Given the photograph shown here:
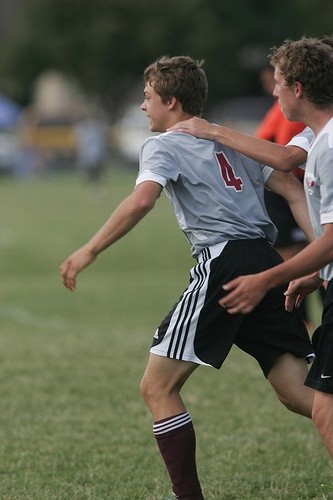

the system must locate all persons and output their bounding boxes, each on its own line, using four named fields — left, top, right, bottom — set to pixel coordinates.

left=59, top=35, right=333, bottom=500
